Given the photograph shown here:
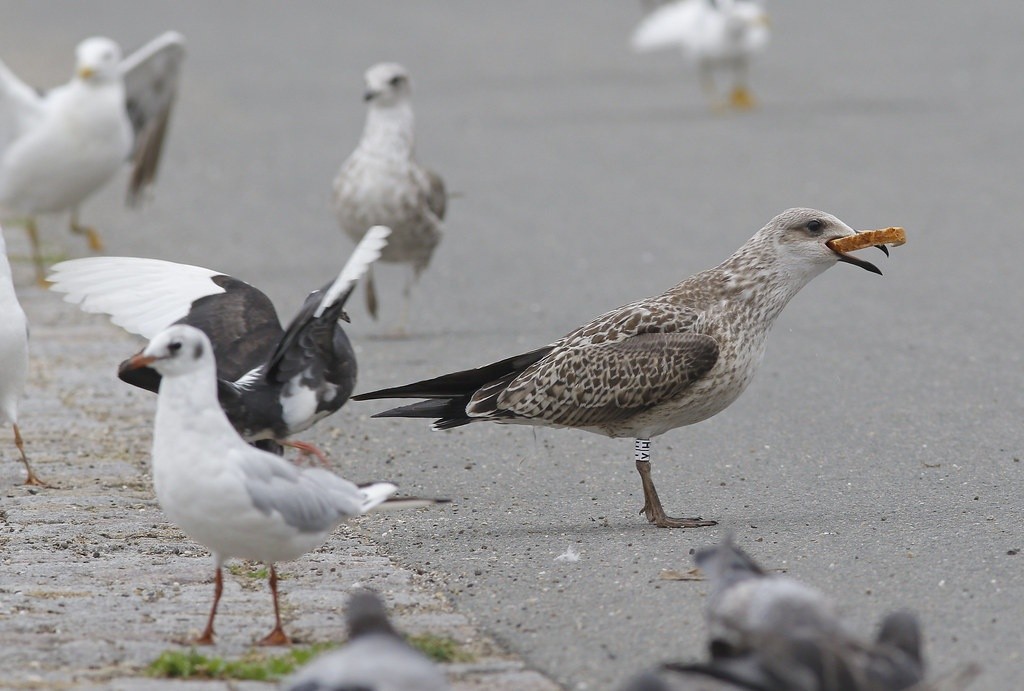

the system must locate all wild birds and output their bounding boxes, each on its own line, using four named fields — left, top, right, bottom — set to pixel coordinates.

left=43, top=223, right=393, bottom=468
left=0, top=28, right=185, bottom=491
left=334, top=60, right=448, bottom=325
left=121, top=322, right=399, bottom=647
left=276, top=535, right=924, bottom=690
left=351, top=205, right=891, bottom=530
left=626, top=0, right=777, bottom=117
